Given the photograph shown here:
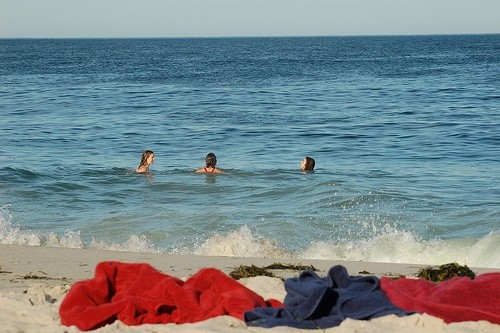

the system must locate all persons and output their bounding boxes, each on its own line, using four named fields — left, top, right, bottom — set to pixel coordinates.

left=135, top=150, right=155, bottom=173
left=300, top=156, right=316, bottom=172
left=196, top=152, right=222, bottom=173
